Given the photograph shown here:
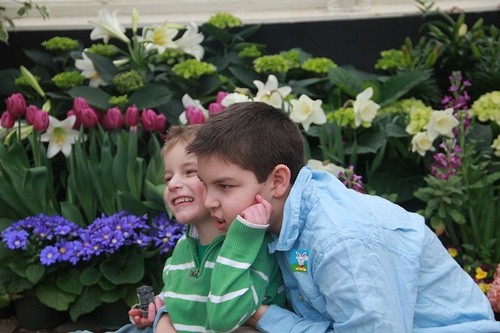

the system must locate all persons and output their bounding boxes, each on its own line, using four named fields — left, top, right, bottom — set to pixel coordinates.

left=152, top=101, right=500, bottom=332
left=114, top=122, right=286, bottom=330
